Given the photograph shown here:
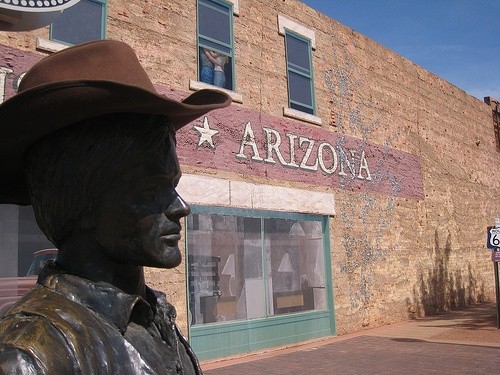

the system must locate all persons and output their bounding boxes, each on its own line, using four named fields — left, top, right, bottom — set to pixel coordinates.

left=200, top=45, right=230, bottom=87
left=0, top=38, right=234, bottom=375
